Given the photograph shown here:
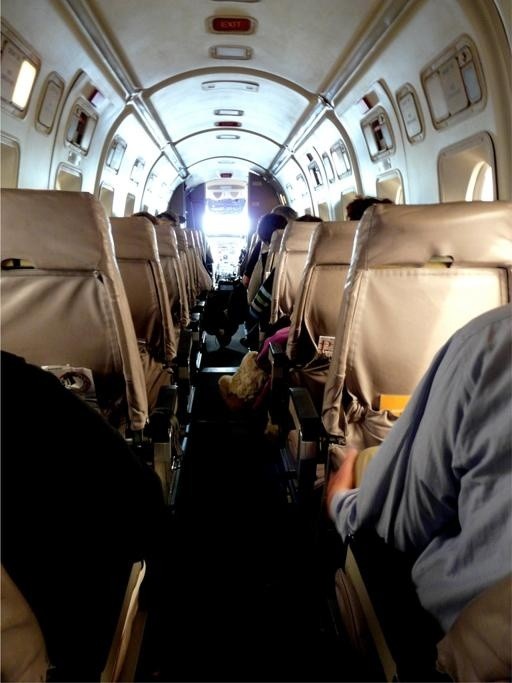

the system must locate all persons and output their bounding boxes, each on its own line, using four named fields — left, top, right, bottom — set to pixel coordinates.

left=325, top=300, right=512, bottom=681
left=239, top=196, right=396, bottom=411
left=132, top=211, right=213, bottom=289
left=1, top=347, right=166, bottom=681
